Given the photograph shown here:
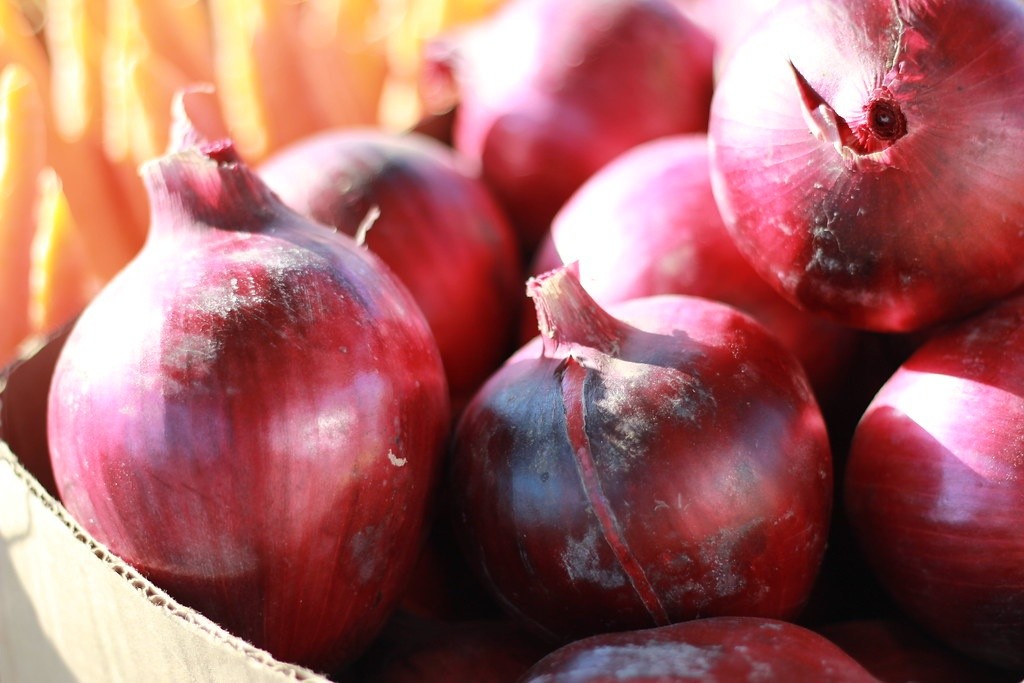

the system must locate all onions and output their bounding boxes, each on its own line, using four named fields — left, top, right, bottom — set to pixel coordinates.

left=44, top=0, right=1024, bottom=683
